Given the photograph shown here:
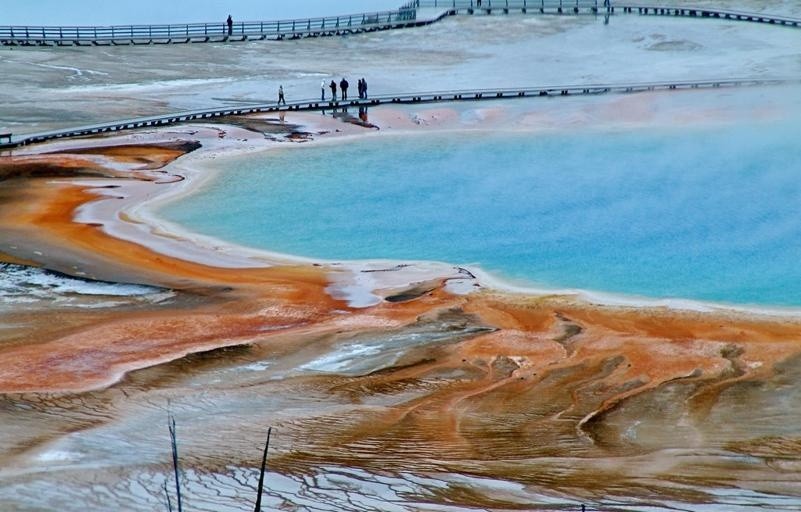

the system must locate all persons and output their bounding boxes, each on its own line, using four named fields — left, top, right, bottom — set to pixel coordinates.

left=322, top=106, right=368, bottom=123
left=320, top=78, right=327, bottom=101
left=277, top=84, right=286, bottom=105
left=329, top=80, right=337, bottom=101
left=227, top=13, right=233, bottom=35
left=603, top=14, right=610, bottom=25
left=358, top=80, right=364, bottom=98
left=360, top=78, right=368, bottom=99
left=340, top=78, right=349, bottom=101
left=604, top=0, right=610, bottom=7
